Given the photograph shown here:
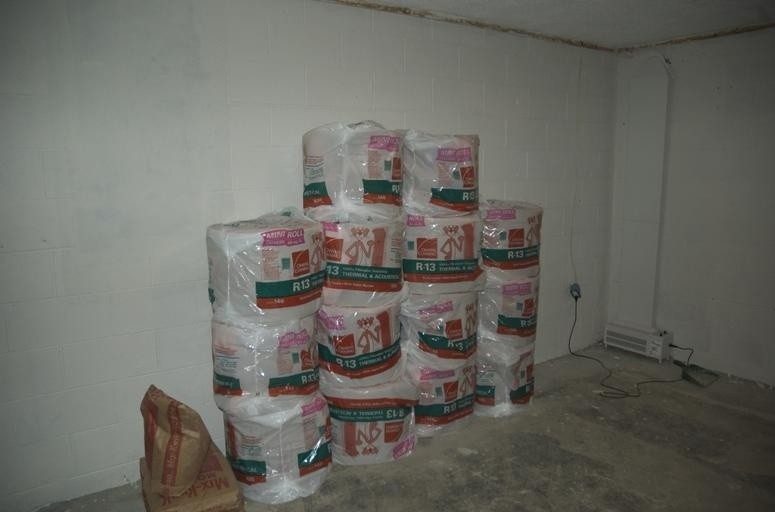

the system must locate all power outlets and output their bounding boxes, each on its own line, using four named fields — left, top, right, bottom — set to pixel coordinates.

left=571, top=283, right=580, bottom=298
